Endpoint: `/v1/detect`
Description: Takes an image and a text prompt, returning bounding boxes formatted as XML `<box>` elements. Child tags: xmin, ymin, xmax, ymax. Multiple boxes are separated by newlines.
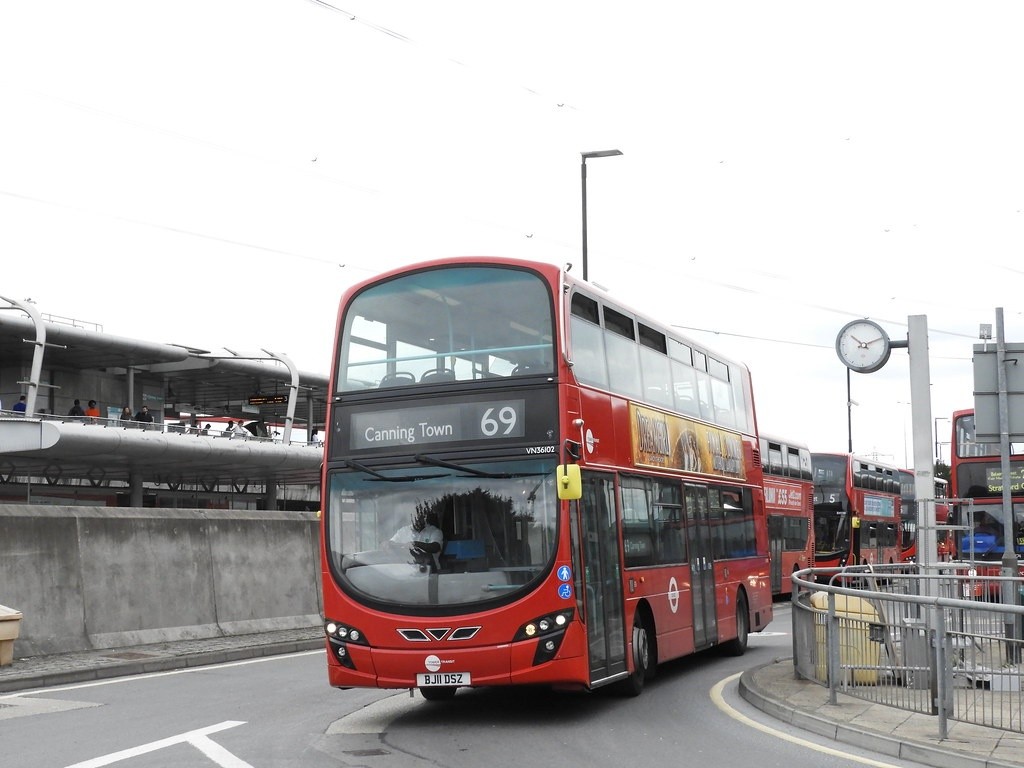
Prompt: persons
<box><xmin>194</xmin><ymin>420</ymin><xmax>248</xmax><ymax>439</ymax></box>
<box><xmin>12</xmin><ymin>397</ymin><xmax>26</xmax><ymax>414</ymax></box>
<box><xmin>379</xmin><ymin>503</ymin><xmax>443</xmax><ymax>571</ymax></box>
<box><xmin>311</xmin><ymin>430</ymin><xmax>320</xmax><ymax>446</ymax></box>
<box><xmin>69</xmin><ymin>399</ymin><xmax>100</xmax><ymax>425</ymax></box>
<box><xmin>120</xmin><ymin>406</ymin><xmax>153</xmax><ymax>429</ymax></box>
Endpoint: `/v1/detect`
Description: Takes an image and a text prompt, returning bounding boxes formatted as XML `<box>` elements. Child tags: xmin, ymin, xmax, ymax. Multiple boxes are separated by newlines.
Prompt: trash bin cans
<box><xmin>811</xmin><ymin>591</ymin><xmax>882</xmax><ymax>686</ymax></box>
<box><xmin>0</xmin><ymin>605</ymin><xmax>23</xmax><ymax>667</ymax></box>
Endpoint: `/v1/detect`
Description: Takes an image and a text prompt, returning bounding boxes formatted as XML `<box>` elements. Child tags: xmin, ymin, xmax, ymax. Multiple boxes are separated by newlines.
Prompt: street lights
<box><xmin>580</xmin><ymin>147</ymin><xmax>623</xmax><ymax>281</ymax></box>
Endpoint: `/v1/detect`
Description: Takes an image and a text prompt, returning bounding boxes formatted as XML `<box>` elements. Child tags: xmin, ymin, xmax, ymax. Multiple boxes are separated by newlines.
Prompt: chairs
<box><xmin>443</xmin><ymin>540</ymin><xmax>486</xmax><ymax>560</ymax></box>
<box><xmin>762</xmin><ymin>463</ymin><xmax>813</xmax><ymax>481</ymax></box>
<box><xmin>377</xmin><ymin>360</ymin><xmax>737</xmax><ymax>432</ymax></box>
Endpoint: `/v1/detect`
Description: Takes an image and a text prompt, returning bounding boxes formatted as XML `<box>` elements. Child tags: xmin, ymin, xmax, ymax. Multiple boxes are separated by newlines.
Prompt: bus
<box><xmin>948</xmin><ymin>409</ymin><xmax>1024</xmax><ymax>596</ymax></box>
<box><xmin>757</xmin><ymin>435</ymin><xmax>817</xmax><ymax>600</ymax></box>
<box><xmin>810</xmin><ymin>451</ymin><xmax>902</xmax><ymax>592</ymax></box>
<box><xmin>318</xmin><ymin>256</ymin><xmax>776</xmax><ymax>711</ymax></box>
<box><xmin>896</xmin><ymin>469</ymin><xmax>957</xmax><ymax>576</ymax></box>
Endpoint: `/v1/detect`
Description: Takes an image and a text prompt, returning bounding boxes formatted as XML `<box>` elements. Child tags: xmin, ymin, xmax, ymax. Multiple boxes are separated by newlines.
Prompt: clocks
<box><xmin>835</xmin><ymin>318</ymin><xmax>891</xmax><ymax>375</ymax></box>
